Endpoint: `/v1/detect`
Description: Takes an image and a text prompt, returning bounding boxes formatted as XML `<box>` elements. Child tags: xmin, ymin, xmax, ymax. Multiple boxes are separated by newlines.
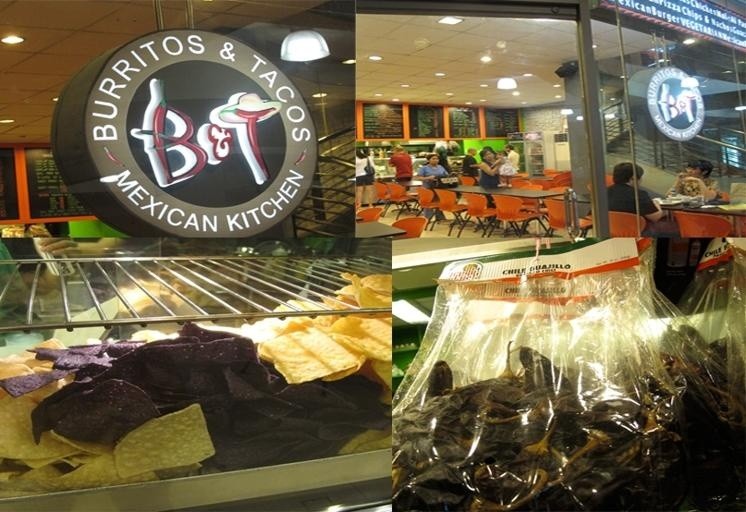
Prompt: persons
<box><xmin>588</xmin><ymin>161</ymin><xmax>674</xmax><ymax>288</ymax></box>
<box><xmin>387</xmin><ymin>144</ymin><xmax>414</xmax><ymax>192</ymax></box>
<box><xmin>461</xmin><ymin>148</ymin><xmax>481</xmax><ymax>177</ymax></box>
<box><xmin>496</xmin><ymin>150</ymin><xmax>517</xmax><ymax>188</ymax></box>
<box><xmin>480</xmin><ymin>147</ymin><xmax>506</xmax><ymax>192</ymax></box>
<box><xmin>414</xmin><ymin>153</ymin><xmax>450</xmax><ymax>226</ymax></box>
<box><xmin>504</xmin><ymin>143</ymin><xmax>520</xmax><ymax>171</ymax></box>
<box><xmin>433</xmin><ymin>137</ymin><xmax>460</xmax><ymax>174</ymax></box>
<box><xmin>665</xmin><ymin>153</ymin><xmax>724</xmax><ymax>207</ymax></box>
<box><xmin>355</xmin><ymin>143</ymin><xmax>376</xmax><ymax>215</ymax></box>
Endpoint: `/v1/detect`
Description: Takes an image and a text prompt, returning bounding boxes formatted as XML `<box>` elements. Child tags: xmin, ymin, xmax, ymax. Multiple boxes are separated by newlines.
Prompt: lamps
<box><xmin>496</xmin><ymin>77</ymin><xmax>518</xmax><ymax>89</ymax></box>
<box><xmin>280</xmin><ymin>30</ymin><xmax>331</xmax><ymax>62</ymax></box>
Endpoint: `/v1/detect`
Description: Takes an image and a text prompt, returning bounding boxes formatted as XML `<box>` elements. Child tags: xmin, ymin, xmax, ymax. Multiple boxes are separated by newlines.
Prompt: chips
<box><xmin>0</xmin><ymin>273</ymin><xmax>392</xmax><ymax>498</ymax></box>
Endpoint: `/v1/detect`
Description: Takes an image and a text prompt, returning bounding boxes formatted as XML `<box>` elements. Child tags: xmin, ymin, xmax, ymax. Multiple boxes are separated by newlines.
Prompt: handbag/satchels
<box><xmin>365</xmin><ymin>166</ymin><xmax>374</xmax><ymax>175</ymax></box>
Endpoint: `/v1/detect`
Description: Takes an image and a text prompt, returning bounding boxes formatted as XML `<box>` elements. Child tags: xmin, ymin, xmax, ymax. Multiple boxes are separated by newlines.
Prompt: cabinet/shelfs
<box><xmin>356</xmin><ymin>144</ymin><xmax>435</xmax><ymax>167</ymax></box>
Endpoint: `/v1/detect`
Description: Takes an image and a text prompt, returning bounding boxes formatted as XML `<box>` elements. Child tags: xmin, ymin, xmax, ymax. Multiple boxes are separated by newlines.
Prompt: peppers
<box><xmin>658</xmin><ymin>93</ymin><xmax>680</xmax><ymax>117</ymax></box>
<box><xmin>295</xmin><ymin>147</ymin><xmax>307</xmax><ymax>165</ymax></box>
<box><xmin>248</xmin><ymin>118</ymin><xmax>269</xmax><ymax>178</ymax></box>
<box><xmin>220</xmin><ymin>106</ymin><xmax>277</xmax><ymax>119</ymax></box>
<box><xmin>104</xmin><ymin>146</ymin><xmax>123</xmax><ymax>165</ymax></box>
<box><xmin>137</xmin><ymin>97</ymin><xmax>208</xmax><ymax>183</ymax></box>
<box><xmin>685</xmin><ymin>95</ymin><xmax>697</xmax><ymax>99</ymax></box>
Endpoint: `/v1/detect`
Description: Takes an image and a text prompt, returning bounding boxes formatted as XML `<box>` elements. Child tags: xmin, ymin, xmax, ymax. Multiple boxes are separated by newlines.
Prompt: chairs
<box><xmin>355</xmin><ymin>169</ymin><xmax>746</xmax><ymax>259</ymax></box>
